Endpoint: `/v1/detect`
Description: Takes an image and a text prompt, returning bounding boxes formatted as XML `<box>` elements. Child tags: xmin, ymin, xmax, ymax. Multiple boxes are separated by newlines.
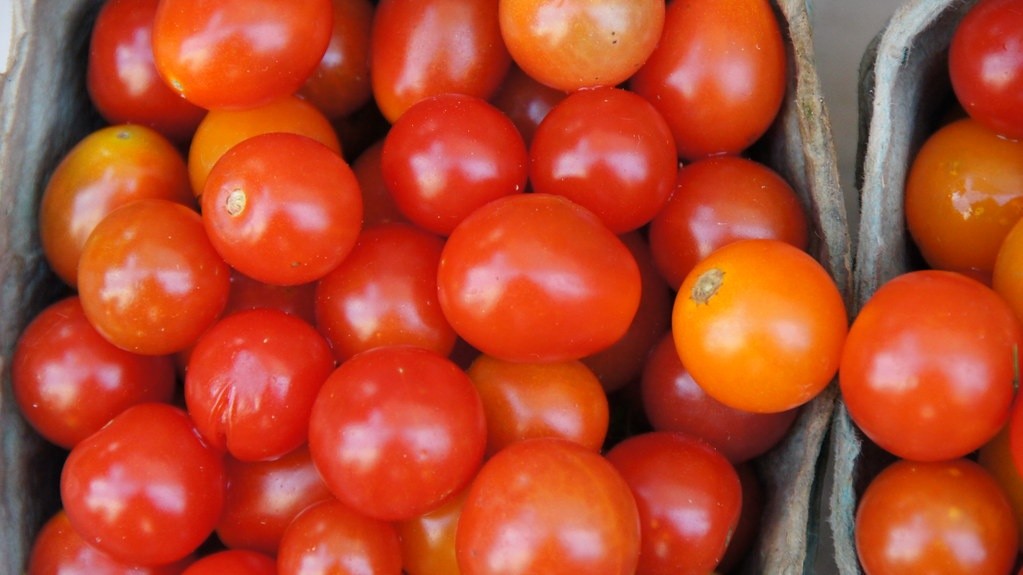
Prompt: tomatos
<box><xmin>0</xmin><ymin>0</ymin><xmax>1023</xmax><ymax>575</ymax></box>
